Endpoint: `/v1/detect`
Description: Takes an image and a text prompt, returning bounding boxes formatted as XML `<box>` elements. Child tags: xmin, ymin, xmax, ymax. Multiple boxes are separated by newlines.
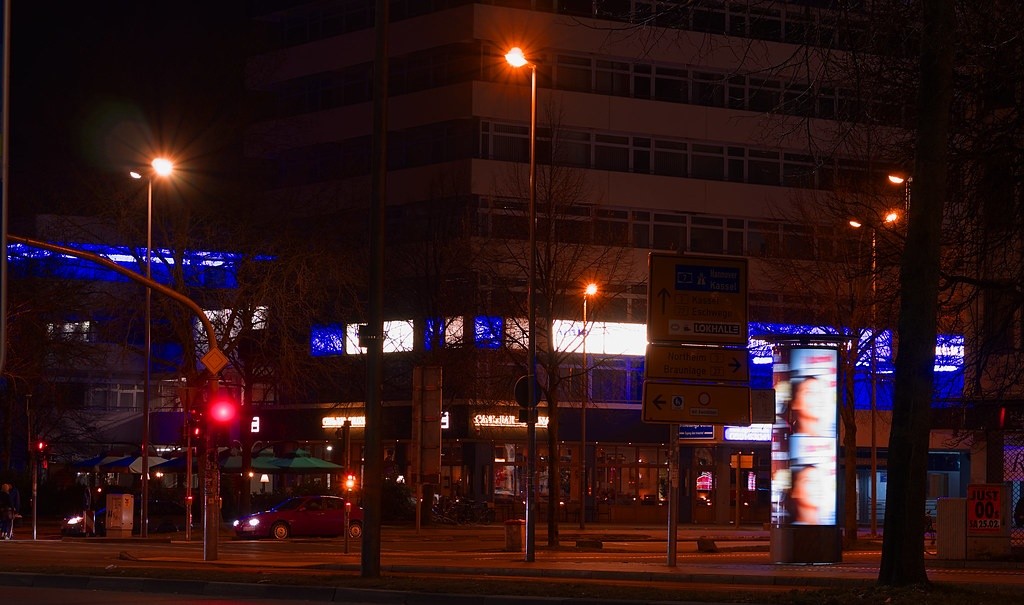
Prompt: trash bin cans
<box><xmin>503</xmin><ymin>519</ymin><xmax>526</xmax><ymax>552</ymax></box>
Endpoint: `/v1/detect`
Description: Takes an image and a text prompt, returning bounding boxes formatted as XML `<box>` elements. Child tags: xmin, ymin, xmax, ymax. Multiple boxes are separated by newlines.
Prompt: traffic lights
<box><xmin>345</xmin><ymin>474</ymin><xmax>356</xmax><ymax>491</ymax></box>
<box><xmin>179</xmin><ymin>386</ymin><xmax>204</xmax><ymax>443</ymax></box>
<box><xmin>204</xmin><ymin>392</ymin><xmax>239</xmax><ymax>454</ymax></box>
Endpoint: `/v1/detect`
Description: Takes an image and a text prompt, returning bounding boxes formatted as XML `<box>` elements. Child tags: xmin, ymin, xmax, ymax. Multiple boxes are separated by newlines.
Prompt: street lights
<box><xmin>139</xmin><ymin>152</ymin><xmax>173</xmax><ymax>537</ymax></box>
<box><xmin>504</xmin><ymin>46</ymin><xmax>538</xmax><ymax>561</ymax></box>
<box><xmin>848</xmin><ymin>219</ymin><xmax>878</xmax><ymax>537</ymax></box>
<box><xmin>31</xmin><ymin>439</ymin><xmax>47</xmax><ymax>539</ymax></box>
<box><xmin>579</xmin><ymin>282</ymin><xmax>597</xmax><ymax>529</ymax></box>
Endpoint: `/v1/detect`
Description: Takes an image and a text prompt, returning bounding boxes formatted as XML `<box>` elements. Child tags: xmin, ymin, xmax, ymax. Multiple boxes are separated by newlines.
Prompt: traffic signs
<box><xmin>642</xmin><ymin>252</ymin><xmax>751</xmax><ymax>425</ymax></box>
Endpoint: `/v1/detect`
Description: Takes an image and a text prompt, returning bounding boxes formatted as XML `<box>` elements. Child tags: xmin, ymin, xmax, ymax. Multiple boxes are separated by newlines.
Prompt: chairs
<box><xmin>487</xmin><ymin>503</ymin><xmax>548</xmax><ymax>523</ymax></box>
<box><xmin>645</xmin><ymin>495</ymin><xmax>656</xmax><ymax>504</ymax></box>
<box><xmin>597</xmin><ymin>503</ymin><xmax>611</xmax><ymax>522</ymax></box>
<box><xmin>565</xmin><ymin>503</ymin><xmax>577</xmax><ymax>522</ymax></box>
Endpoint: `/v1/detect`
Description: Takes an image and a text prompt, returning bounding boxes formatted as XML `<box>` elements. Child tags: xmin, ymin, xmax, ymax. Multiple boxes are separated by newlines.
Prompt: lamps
<box><xmin>260</xmin><ymin>474</ymin><xmax>269</xmax><ymax>492</ymax></box>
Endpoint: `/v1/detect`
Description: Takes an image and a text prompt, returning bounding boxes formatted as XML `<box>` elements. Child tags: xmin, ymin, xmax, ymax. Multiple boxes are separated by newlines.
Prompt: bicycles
<box><xmin>924</xmin><ymin>509</ymin><xmax>937</xmax><ymax>555</ymax></box>
<box><xmin>431</xmin><ymin>496</ymin><xmax>496</xmax><ymax>526</ymax></box>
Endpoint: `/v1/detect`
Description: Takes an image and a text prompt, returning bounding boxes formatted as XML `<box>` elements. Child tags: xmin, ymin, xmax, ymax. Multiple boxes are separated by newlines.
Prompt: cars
<box><xmin>232</xmin><ymin>495</ymin><xmax>363</xmax><ymax>540</ymax></box>
<box><xmin>61</xmin><ymin>498</ymin><xmax>193</xmax><ymax>536</ymax></box>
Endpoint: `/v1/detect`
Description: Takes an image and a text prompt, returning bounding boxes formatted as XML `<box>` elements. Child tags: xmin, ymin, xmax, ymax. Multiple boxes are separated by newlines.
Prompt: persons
<box><xmin>0</xmin><ymin>483</ymin><xmax>20</xmax><ymax>539</ymax></box>
